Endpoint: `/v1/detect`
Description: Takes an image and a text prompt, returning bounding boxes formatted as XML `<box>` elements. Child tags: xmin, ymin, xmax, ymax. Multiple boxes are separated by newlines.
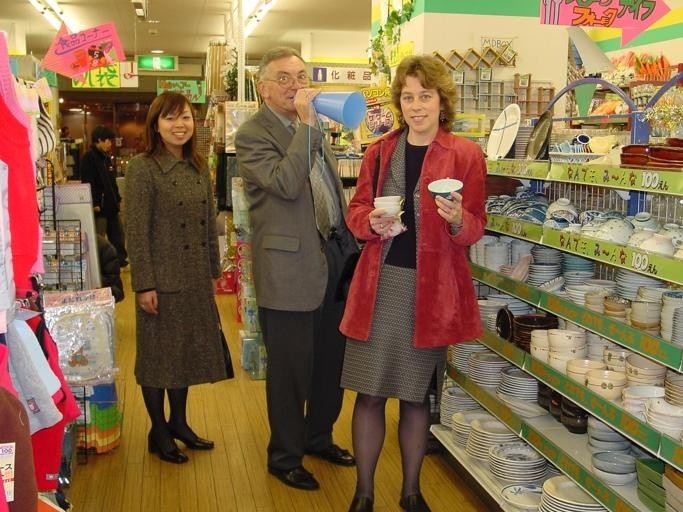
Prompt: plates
<box><xmin>515</xmin><ymin>126</ymin><xmax>534</xmax><ymax>159</ymax></box>
<box><xmin>439</xmin><ymin>339</ymin><xmax>604</xmax><ymax>512</ymax></box>
<box><xmin>553</xmin><ymin>140</ymin><xmax>592</xmax><ymax>152</ymax></box>
<box><xmin>478</xmin><ymin>238</ymin><xmax>682</xmax><ymax>346</ymax></box>
<box><xmin>486</xmin><ymin>104</ymin><xmax>521</xmax><ymax>160</ymax></box>
<box><xmin>526</xmin><ymin>111</ymin><xmax>552</xmax><ymax>161</ymax></box>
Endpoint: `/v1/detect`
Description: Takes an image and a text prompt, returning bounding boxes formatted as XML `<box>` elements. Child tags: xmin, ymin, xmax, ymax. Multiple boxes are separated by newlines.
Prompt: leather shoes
<box><xmin>348</xmin><ymin>496</ymin><xmax>374</xmax><ymax>512</ymax></box>
<box><xmin>307</xmin><ymin>442</ymin><xmax>355</xmax><ymax>466</ymax></box>
<box><xmin>269</xmin><ymin>464</ymin><xmax>319</xmax><ymax>490</ymax></box>
<box><xmin>399</xmin><ymin>493</ymin><xmax>430</xmax><ymax>511</ymax></box>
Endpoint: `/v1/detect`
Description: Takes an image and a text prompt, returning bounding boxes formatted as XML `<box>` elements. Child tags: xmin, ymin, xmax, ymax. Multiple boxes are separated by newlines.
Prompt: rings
<box><xmin>141</xmin><ymin>305</ymin><xmax>144</xmax><ymax>308</ymax></box>
<box><xmin>381</xmin><ymin>224</ymin><xmax>383</xmax><ymax>229</ymax></box>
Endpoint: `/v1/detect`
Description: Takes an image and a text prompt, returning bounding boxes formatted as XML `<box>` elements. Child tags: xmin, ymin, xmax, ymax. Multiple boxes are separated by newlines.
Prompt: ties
<box><xmin>291</xmin><ymin>120</ymin><xmax>331</xmax><ymax>241</ymax></box>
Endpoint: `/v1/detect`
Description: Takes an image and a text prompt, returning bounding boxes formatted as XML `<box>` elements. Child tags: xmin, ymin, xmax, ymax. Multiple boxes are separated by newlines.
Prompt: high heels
<box><xmin>173</xmin><ymin>425</ymin><xmax>214</xmax><ymax>450</ymax></box>
<box><xmin>148</xmin><ymin>435</ymin><xmax>189</xmax><ymax>464</ymax></box>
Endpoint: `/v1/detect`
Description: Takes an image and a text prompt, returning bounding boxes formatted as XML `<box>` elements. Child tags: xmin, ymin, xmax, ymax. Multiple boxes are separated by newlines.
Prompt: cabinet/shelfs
<box><xmin>429</xmin><ymin>108</ymin><xmax>683</xmax><ymax>511</ymax></box>
<box><xmin>441</xmin><ymin>68</ymin><xmax>515</xmax><ymax>113</ymax></box>
<box><xmin>515</xmin><ymin>74</ymin><xmax>555</xmax><ymax>118</ymax></box>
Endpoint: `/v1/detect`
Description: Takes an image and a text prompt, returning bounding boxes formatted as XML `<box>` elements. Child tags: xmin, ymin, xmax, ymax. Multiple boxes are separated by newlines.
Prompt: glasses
<box><xmin>265</xmin><ymin>77</ymin><xmax>312</xmax><ymax>90</ymax></box>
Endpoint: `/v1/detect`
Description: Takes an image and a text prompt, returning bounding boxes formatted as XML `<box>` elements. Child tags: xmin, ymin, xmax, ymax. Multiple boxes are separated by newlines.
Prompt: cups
<box><xmin>571</xmin><ymin>134</ymin><xmax>591</xmax><ymax>146</ymax></box>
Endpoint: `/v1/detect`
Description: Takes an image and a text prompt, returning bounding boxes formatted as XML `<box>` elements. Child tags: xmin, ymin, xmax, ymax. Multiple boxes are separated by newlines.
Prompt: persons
<box><xmin>80</xmin><ymin>128</ymin><xmax>128</xmax><ymax>268</ymax></box>
<box><xmin>340</xmin><ymin>55</ymin><xmax>487</xmax><ymax>512</ymax></box>
<box><xmin>98</xmin><ymin>236</ymin><xmax>124</xmax><ymax>303</ymax></box>
<box><xmin>235</xmin><ymin>47</ymin><xmax>355</xmax><ymax>489</ymax></box>
<box><xmin>124</xmin><ymin>92</ymin><xmax>233</xmax><ymax>464</ymax></box>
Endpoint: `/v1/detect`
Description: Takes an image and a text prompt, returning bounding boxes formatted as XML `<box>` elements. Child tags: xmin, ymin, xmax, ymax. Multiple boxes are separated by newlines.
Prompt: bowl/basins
<box><xmin>483</xmin><ymin>185</ymin><xmax>682</xmax><ymax>261</ymax></box>
<box><xmin>620</xmin><ymin>138</ymin><xmax>683</xmax><ymax>167</ymax></box>
<box><xmin>428</xmin><ymin>178</ymin><xmax>464</xmax><ymax>201</ymax></box>
<box><xmin>478</xmin><ymin>226</ymin><xmax>683</xmax><ymax>348</ymax></box>
<box><xmin>373</xmin><ymin>196</ymin><xmax>401</xmax><ymax>218</ymax></box>
<box><xmin>529</xmin><ymin>305</ymin><xmax>683</xmax><ymax>511</ymax></box>
